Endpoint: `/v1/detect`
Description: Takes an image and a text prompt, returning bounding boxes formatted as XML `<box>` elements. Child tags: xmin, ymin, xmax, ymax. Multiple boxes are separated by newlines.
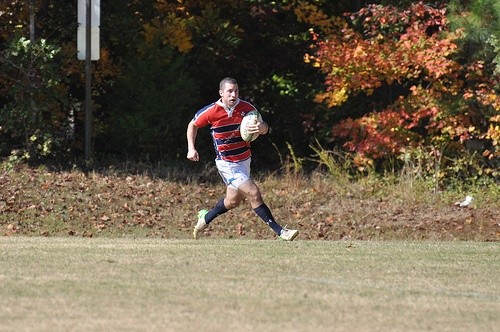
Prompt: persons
<box><xmin>185</xmin><ymin>77</ymin><xmax>299</xmax><ymax>242</ymax></box>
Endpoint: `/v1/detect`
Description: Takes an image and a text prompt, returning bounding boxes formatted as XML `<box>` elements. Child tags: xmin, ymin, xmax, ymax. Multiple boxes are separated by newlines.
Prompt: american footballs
<box><xmin>239</xmin><ymin>110</ymin><xmax>263</xmax><ymax>142</ymax></box>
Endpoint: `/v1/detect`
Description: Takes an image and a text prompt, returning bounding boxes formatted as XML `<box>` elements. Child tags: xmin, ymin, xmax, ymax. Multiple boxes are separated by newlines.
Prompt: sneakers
<box><xmin>277</xmin><ymin>226</ymin><xmax>299</xmax><ymax>241</ymax></box>
<box><xmin>193</xmin><ymin>209</ymin><xmax>209</xmax><ymax>239</ymax></box>
